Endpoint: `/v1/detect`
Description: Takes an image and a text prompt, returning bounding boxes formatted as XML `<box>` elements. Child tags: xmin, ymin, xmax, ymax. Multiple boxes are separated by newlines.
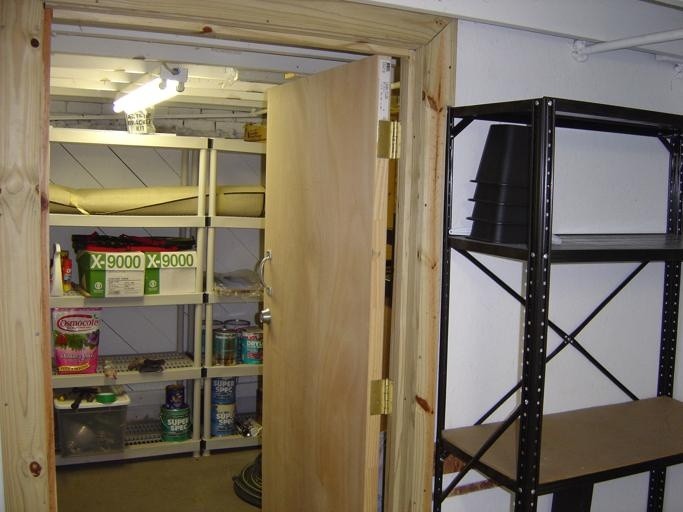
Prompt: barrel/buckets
<box><xmin>213</xmin><ymin>329</ymin><xmax>240</xmax><ymax>366</ymax></box>
<box><xmin>201</xmin><ymin>319</ymin><xmax>224</xmax><ymax>359</ymax></box>
<box><xmin>211</xmin><ymin>404</ymin><xmax>237</xmax><ymax>436</ymax></box>
<box><xmin>240</xmin><ymin>326</ymin><xmax>263</xmax><ymax>364</ymax></box>
<box><xmin>212</xmin><ymin>377</ymin><xmax>238</xmax><ymax>405</ymax></box>
<box><xmin>224</xmin><ymin>319</ymin><xmax>249</xmax><ymax>360</ymax></box>
<box><xmin>160</xmin><ymin>404</ymin><xmax>193</xmax><ymax>443</ymax></box>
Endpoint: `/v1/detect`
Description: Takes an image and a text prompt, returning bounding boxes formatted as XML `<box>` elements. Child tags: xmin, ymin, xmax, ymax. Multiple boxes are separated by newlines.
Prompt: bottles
<box><xmin>62</xmin><ymin>259</ymin><xmax>72</xmax><ymax>293</ymax></box>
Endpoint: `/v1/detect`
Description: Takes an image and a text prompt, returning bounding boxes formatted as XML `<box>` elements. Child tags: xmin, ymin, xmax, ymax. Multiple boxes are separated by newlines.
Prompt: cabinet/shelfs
<box><xmin>435</xmin><ymin>95</ymin><xmax>682</xmax><ymax>512</ymax></box>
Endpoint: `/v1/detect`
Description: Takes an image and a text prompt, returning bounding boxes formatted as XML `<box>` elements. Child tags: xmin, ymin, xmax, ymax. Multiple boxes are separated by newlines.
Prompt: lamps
<box><xmin>114</xmin><ymin>65</ymin><xmax>188</xmax><ymax>114</ymax></box>
